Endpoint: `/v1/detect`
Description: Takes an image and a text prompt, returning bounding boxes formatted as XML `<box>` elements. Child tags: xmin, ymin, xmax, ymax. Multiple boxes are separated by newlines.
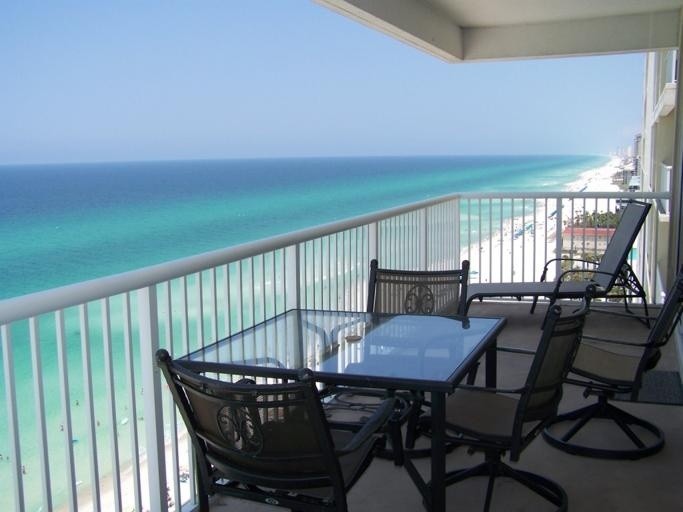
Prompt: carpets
<box><xmin>612</xmin><ymin>371</ymin><xmax>683</xmax><ymax>405</ymax></box>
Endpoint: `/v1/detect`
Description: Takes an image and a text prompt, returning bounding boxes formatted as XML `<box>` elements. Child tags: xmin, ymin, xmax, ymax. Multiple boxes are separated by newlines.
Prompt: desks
<box><xmin>174</xmin><ymin>308</ymin><xmax>507</xmax><ymax>512</ymax></box>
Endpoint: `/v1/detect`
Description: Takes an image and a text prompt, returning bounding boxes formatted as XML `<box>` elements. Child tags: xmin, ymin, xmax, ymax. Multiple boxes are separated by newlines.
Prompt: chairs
<box><xmin>540</xmin><ymin>264</ymin><xmax>683</xmax><ymax>457</ymax></box>
<box><xmin>462</xmin><ymin>198</ymin><xmax>654</xmax><ymax>329</ymax></box>
<box><xmin>365</xmin><ymin>258</ymin><xmax>482</xmax><ymax>452</ymax></box>
<box><xmin>156</xmin><ymin>350</ymin><xmax>401</xmax><ymax>512</ymax></box>
<box><xmin>418</xmin><ymin>283</ymin><xmax>596</xmax><ymax>512</ymax></box>
